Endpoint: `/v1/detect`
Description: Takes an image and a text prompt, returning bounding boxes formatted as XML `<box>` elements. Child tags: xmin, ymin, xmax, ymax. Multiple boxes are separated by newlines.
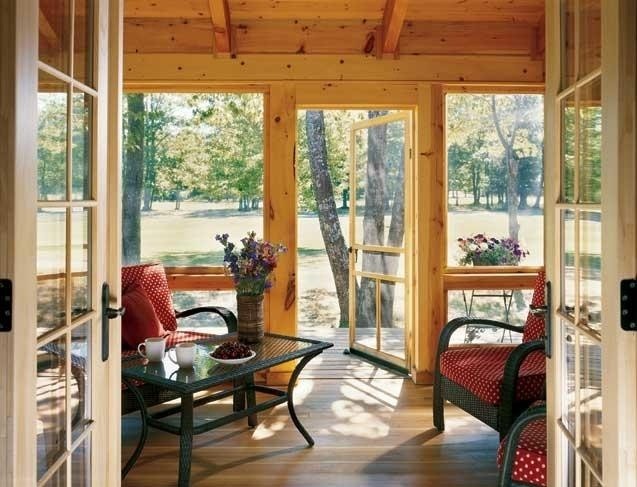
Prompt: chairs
<box><xmin>122</xmin><ymin>263</ymin><xmax>236</xmax><ymax>416</ymax></box>
<box><xmin>433</xmin><ymin>268</ymin><xmax>547</xmax><ymax>439</ymax></box>
<box><xmin>496</xmin><ymin>399</ymin><xmax>548</xmax><ymax>487</ymax></box>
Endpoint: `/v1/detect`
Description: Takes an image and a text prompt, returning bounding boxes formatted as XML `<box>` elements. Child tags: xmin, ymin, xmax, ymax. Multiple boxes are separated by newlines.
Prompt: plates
<box><xmin>207</xmin><ymin>350</ymin><xmax>256</xmax><ymax>365</ymax></box>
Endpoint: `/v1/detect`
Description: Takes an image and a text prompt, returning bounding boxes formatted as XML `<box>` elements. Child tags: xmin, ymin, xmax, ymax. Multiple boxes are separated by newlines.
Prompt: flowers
<box><xmin>215</xmin><ymin>232</ymin><xmax>288</xmax><ymax>296</ymax></box>
<box><xmin>457</xmin><ymin>232</ymin><xmax>531</xmax><ymax>266</ymax></box>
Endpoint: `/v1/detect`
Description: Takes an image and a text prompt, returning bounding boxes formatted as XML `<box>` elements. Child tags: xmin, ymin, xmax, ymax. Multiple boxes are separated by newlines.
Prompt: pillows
<box><xmin>124</xmin><ymin>283</ymin><xmax>163</xmax><ymax>346</ymax></box>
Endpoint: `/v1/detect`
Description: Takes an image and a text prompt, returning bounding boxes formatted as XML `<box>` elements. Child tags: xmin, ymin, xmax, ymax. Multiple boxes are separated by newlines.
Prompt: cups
<box><xmin>137</xmin><ymin>337</ymin><xmax>166</xmax><ymax>362</ymax></box>
<box><xmin>168</xmin><ymin>342</ymin><xmax>197</xmax><ymax>369</ymax></box>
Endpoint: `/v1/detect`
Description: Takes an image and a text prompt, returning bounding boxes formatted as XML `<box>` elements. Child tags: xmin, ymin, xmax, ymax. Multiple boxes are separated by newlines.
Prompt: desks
<box><xmin>121</xmin><ymin>333</ymin><xmax>334</xmax><ymax>487</ymax></box>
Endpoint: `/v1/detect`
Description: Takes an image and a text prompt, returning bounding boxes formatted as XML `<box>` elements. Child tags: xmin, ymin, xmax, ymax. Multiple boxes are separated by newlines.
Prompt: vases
<box><xmin>472</xmin><ymin>258</ymin><xmax>506</xmax><ymax>266</ymax></box>
<box><xmin>235</xmin><ymin>295</ymin><xmax>265</xmax><ymax>344</ymax></box>
